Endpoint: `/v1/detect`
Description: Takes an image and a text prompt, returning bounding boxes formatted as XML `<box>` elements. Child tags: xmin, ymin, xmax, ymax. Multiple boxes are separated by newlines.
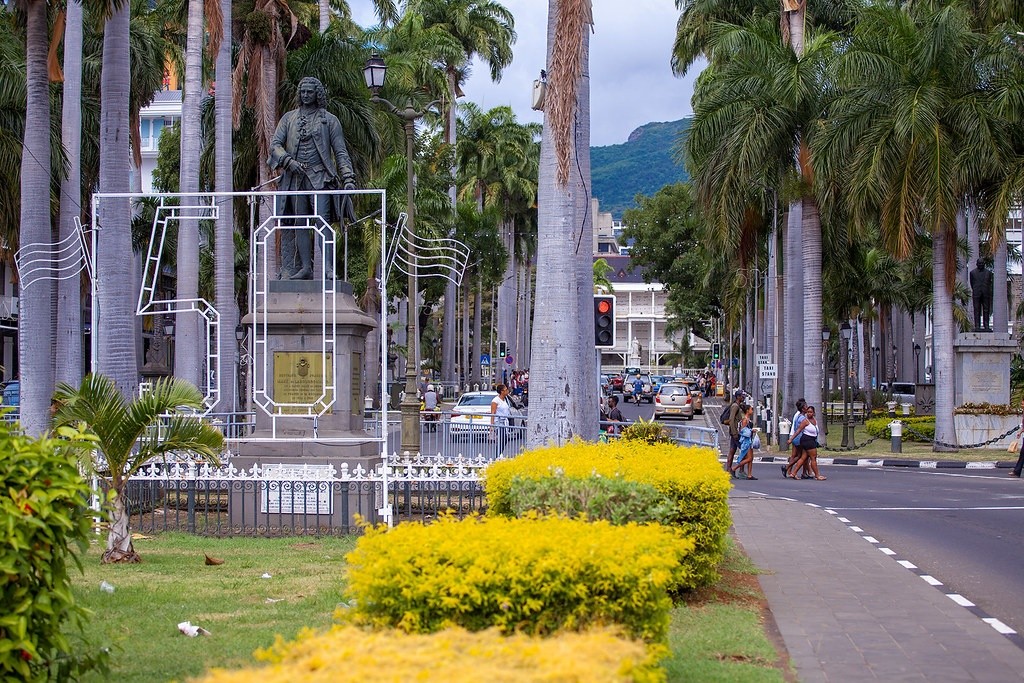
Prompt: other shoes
<box><xmin>634</xmin><ymin>400</ymin><xmax>636</xmax><ymax>404</ymax></box>
<box><xmin>802</xmin><ymin>474</ymin><xmax>813</xmax><ymax>479</ymax></box>
<box><xmin>781</xmin><ymin>465</ymin><xmax>787</xmax><ymax>478</ymax></box>
<box><xmin>748</xmin><ymin>476</ymin><xmax>758</xmax><ymax>480</ymax></box>
<box><xmin>736</xmin><ymin>471</ymin><xmax>747</xmax><ymax>479</ymax></box>
<box><xmin>1008</xmin><ymin>472</ymin><xmax>1020</xmax><ymax>477</ymax></box>
<box><xmin>729</xmin><ymin>468</ymin><xmax>737</xmax><ymax>479</ymax></box>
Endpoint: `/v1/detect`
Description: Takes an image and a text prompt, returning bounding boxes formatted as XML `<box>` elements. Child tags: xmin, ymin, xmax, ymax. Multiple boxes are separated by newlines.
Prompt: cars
<box><xmin>449</xmin><ymin>391</ymin><xmax>526</xmax><ymax>443</ymax></box>
<box><xmin>600</xmin><ymin>373</ymin><xmax>676</xmax><ymax>396</ymax></box>
<box><xmin>654</xmin><ymin>383</ymin><xmax>693</xmax><ymax>421</ymax></box>
<box><xmin>672</xmin><ymin>380</ymin><xmax>703</xmax><ymax>415</ymax></box>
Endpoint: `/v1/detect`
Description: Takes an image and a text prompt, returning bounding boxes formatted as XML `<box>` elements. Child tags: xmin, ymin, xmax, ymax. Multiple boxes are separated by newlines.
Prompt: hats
<box><xmin>735</xmin><ymin>390</ymin><xmax>747</xmax><ymax>396</ymax></box>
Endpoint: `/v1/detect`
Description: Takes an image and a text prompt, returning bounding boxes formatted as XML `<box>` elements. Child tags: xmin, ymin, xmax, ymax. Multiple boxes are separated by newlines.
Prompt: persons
<box><xmin>500</xmin><ymin>369</ymin><xmax>530</xmax><ymax>388</ymax></box>
<box><xmin>632</xmin><ymin>374</ymin><xmax>644</xmax><ymax>405</ymax></box>
<box><xmin>1008</xmin><ymin>410</ymin><xmax>1024</xmax><ymax>477</ymax></box>
<box><xmin>685</xmin><ymin>372</ymin><xmax>690</xmax><ymax>379</ymax></box>
<box><xmin>781</xmin><ymin>398</ymin><xmax>816</xmax><ymax>479</ymax></box>
<box><xmin>437</xmin><ymin>383</ymin><xmax>444</xmax><ymax>400</ymax></box>
<box><xmin>787</xmin><ymin>406</ymin><xmax>828</xmax><ymax>480</ymax></box>
<box><xmin>268</xmin><ymin>74</ymin><xmax>356</xmax><ymax>280</ymax></box>
<box><xmin>399</xmin><ymin>389</ymin><xmax>407</xmax><ymax>402</ymax></box>
<box><xmin>415</xmin><ymin>388</ymin><xmax>421</xmax><ymax>401</ymax></box>
<box><xmin>693</xmin><ymin>370</ymin><xmax>716</xmax><ymax>398</ymax></box>
<box><xmin>969</xmin><ymin>258</ymin><xmax>994</xmax><ymax>329</ymax></box>
<box><xmin>728</xmin><ymin>404</ymin><xmax>759</xmax><ymax>480</ymax></box>
<box><xmin>423</xmin><ymin>384</ymin><xmax>441</xmax><ymax>422</ymax></box>
<box><xmin>606</xmin><ymin>395</ymin><xmax>624</xmax><ymax>434</ymax></box>
<box><xmin>727</xmin><ymin>390</ymin><xmax>749</xmax><ymax>476</ymax></box>
<box><xmin>491</xmin><ymin>384</ymin><xmax>510</xmax><ymax>458</ymax></box>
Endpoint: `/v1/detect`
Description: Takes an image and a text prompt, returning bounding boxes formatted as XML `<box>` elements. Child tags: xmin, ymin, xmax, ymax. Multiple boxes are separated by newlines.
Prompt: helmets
<box><xmin>636</xmin><ymin>374</ymin><xmax>641</xmax><ymax>379</ymax></box>
<box><xmin>427</xmin><ymin>385</ymin><xmax>434</xmax><ymax>392</ymax></box>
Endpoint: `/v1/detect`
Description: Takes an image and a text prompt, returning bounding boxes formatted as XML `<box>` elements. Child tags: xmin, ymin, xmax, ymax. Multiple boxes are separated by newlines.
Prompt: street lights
<box><xmin>892</xmin><ymin>345</ymin><xmax>897</xmax><ymax>381</ymax></box>
<box><xmin>913</xmin><ymin>344</ymin><xmax>921</xmax><ymax>384</ymax></box>
<box><xmin>361</xmin><ymin>48</ymin><xmax>449</xmax><ymax>476</ymax></box>
<box><xmin>874</xmin><ymin>347</ymin><xmax>880</xmax><ymax>391</ymax></box>
<box><xmin>821</xmin><ymin>324</ymin><xmax>831</xmax><ymax>435</ymax></box>
<box><xmin>432</xmin><ymin>338</ymin><xmax>437</xmax><ymax>381</ymax></box>
<box><xmin>840</xmin><ymin>321</ymin><xmax>851</xmax><ymax>448</ymax></box>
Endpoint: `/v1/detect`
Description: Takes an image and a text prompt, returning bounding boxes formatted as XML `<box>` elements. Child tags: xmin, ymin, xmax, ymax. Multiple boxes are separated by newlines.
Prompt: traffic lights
<box><xmin>499</xmin><ymin>341</ymin><xmax>506</xmax><ymax>359</ymax></box>
<box><xmin>712</xmin><ymin>343</ymin><xmax>721</xmax><ymax>362</ymax></box>
<box><xmin>507</xmin><ymin>348</ymin><xmax>510</xmax><ymax>357</ymax></box>
<box><xmin>592</xmin><ymin>294</ymin><xmax>617</xmax><ymax>349</ymax></box>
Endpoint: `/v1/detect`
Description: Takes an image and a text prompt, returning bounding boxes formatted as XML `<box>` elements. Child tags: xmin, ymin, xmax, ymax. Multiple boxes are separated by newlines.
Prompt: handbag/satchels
<box><xmin>1007</xmin><ymin>438</ymin><xmax>1019</xmax><ymax>453</ymax></box>
<box><xmin>753</xmin><ymin>431</ymin><xmax>761</xmax><ymax>449</ymax></box>
<box><xmin>720</xmin><ymin>402</ymin><xmax>739</xmax><ymax>424</ymax></box>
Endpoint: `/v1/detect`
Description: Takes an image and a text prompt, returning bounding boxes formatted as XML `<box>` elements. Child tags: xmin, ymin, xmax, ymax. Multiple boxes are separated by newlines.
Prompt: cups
<box><xmin>100</xmin><ymin>580</ymin><xmax>114</xmax><ymax>593</ymax></box>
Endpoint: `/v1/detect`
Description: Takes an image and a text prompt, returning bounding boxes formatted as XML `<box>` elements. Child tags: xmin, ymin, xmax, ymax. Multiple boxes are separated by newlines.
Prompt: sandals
<box><xmin>815</xmin><ymin>476</ymin><xmax>827</xmax><ymax>480</ymax></box>
<box><xmin>790</xmin><ymin>473</ymin><xmax>801</xmax><ymax>480</ymax></box>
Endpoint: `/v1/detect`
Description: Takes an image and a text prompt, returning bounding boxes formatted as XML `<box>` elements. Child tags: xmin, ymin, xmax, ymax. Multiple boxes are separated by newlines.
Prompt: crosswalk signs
<box><xmin>480</xmin><ymin>354</ymin><xmax>489</xmax><ymax>365</ymax></box>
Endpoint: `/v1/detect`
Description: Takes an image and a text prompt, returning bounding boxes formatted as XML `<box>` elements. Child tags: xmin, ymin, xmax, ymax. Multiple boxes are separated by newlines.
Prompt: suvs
<box><xmin>423</xmin><ymin>402</ymin><xmax>443</xmax><ymax>433</ymax></box>
<box><xmin>622</xmin><ymin>374</ymin><xmax>656</xmax><ymax>404</ymax></box>
